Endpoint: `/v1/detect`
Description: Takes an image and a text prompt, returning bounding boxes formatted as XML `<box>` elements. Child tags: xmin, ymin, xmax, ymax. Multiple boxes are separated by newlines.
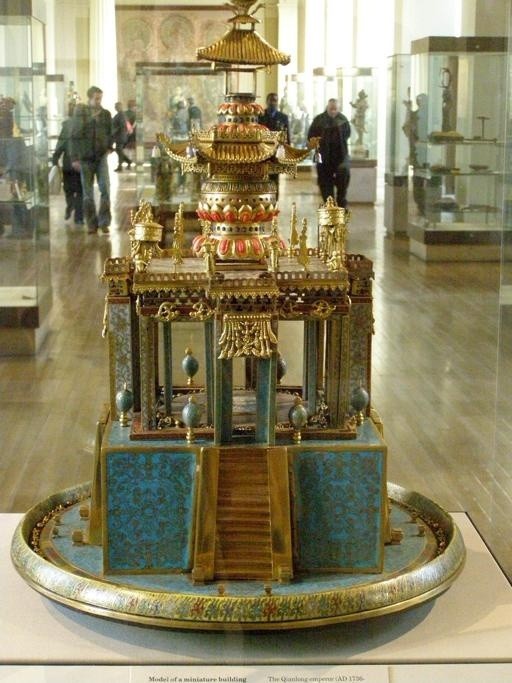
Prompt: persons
<box><xmin>66</xmin><ymin>84</ymin><xmax>116</xmax><ymax>236</ymax></box>
<box><xmin>185</xmin><ymin>96</ymin><xmax>202</xmax><ymax>133</ymax></box>
<box><xmin>400</xmin><ymin>92</ymin><xmax>434</xmax><ymax>214</ymax></box>
<box><xmin>35</xmin><ymin>107</ymin><xmax>49</xmax><ymax>154</ymax></box>
<box><xmin>307</xmin><ymin>97</ymin><xmax>352</xmax><ymax>211</ymax></box>
<box><xmin>172</xmin><ymin>100</ymin><xmax>190</xmax><ymax>136</ymax></box>
<box><xmin>257</xmin><ymin>91</ymin><xmax>290</xmax><ymax>151</ymax></box>
<box><xmin>123</xmin><ymin>99</ymin><xmax>138</xmax><ymax>147</ymax></box>
<box><xmin>0</xmin><ymin>96</ymin><xmax>17</xmax><ymax>138</ymax></box>
<box><xmin>348</xmin><ymin>89</ymin><xmax>369</xmax><ymax>145</ymax></box>
<box><xmin>51</xmin><ymin>101</ymin><xmax>86</xmax><ymax>227</ymax></box>
<box><xmin>107</xmin><ymin>101</ymin><xmax>133</xmax><ymax>172</ymax></box>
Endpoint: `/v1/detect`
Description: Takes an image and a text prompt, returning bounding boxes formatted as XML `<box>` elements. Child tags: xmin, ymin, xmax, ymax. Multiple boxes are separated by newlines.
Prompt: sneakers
<box><xmin>115</xmin><ymin>163</ymin><xmax>131</xmax><ymax>171</ymax></box>
<box><xmin>65</xmin><ymin>212</ymin><xmax>84</xmax><ymax>225</ymax></box>
<box><xmin>88</xmin><ymin>225</ymin><xmax>109</xmax><ymax>233</ymax></box>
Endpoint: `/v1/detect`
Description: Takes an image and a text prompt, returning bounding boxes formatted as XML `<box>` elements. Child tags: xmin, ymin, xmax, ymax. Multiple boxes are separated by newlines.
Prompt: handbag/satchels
<box><xmin>47</xmin><ymin>164</ymin><xmax>61</xmax><ymax>195</ymax></box>
